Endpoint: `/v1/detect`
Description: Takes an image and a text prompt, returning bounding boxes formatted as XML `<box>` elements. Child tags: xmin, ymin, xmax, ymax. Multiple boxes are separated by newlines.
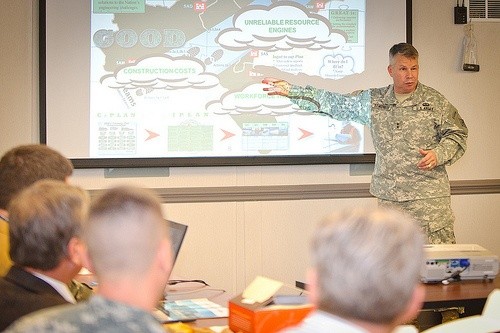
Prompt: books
<box><xmin>152</xmin><ymin>298</ymin><xmax>229</xmax><ymax>323</ymax></box>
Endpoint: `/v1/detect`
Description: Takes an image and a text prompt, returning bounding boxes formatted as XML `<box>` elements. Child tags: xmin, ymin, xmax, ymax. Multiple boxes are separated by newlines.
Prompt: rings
<box><xmin>429</xmin><ymin>166</ymin><xmax>433</xmax><ymax>170</ymax></box>
<box><xmin>428</xmin><ymin>163</ymin><xmax>431</xmax><ymax>167</ymax></box>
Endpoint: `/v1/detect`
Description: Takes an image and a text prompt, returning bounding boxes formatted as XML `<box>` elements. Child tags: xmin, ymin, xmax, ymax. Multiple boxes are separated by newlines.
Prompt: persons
<box><xmin>277</xmin><ymin>203</ymin><xmax>428</xmax><ymax>333</ymax></box>
<box><xmin>0</xmin><ymin>179</ymin><xmax>91</xmax><ymax>333</ymax></box>
<box><xmin>1</xmin><ymin>184</ymin><xmax>175</xmax><ymax>333</ymax></box>
<box><xmin>262</xmin><ymin>42</ymin><xmax>468</xmax><ymax>244</ymax></box>
<box><xmin>0</xmin><ymin>145</ymin><xmax>73</xmax><ymax>276</ymax></box>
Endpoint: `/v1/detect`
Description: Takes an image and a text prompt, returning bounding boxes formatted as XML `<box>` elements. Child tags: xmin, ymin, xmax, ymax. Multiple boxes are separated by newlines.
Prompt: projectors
<box><xmin>421</xmin><ymin>244</ymin><xmax>499</xmax><ymax>285</ymax></box>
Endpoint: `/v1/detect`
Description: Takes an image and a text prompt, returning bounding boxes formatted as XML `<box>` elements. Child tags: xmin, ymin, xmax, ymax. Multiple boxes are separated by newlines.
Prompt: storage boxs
<box><xmin>228</xmin><ymin>275</ymin><xmax>316</xmax><ymax>333</ymax></box>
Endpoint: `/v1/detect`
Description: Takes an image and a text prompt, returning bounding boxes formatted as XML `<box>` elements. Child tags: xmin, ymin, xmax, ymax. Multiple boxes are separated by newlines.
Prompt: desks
<box><xmin>73</xmin><ymin>272</ymin><xmax>232</xmax><ymax>327</ymax></box>
<box><xmin>296</xmin><ymin>279</ymin><xmax>500</xmax><ymax>323</ymax></box>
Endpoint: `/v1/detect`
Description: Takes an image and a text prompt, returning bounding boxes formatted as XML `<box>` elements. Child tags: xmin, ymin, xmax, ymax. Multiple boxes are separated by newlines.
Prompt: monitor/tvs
<box><xmin>164</xmin><ymin>218</ymin><xmax>188</xmax><ymax>264</ymax></box>
